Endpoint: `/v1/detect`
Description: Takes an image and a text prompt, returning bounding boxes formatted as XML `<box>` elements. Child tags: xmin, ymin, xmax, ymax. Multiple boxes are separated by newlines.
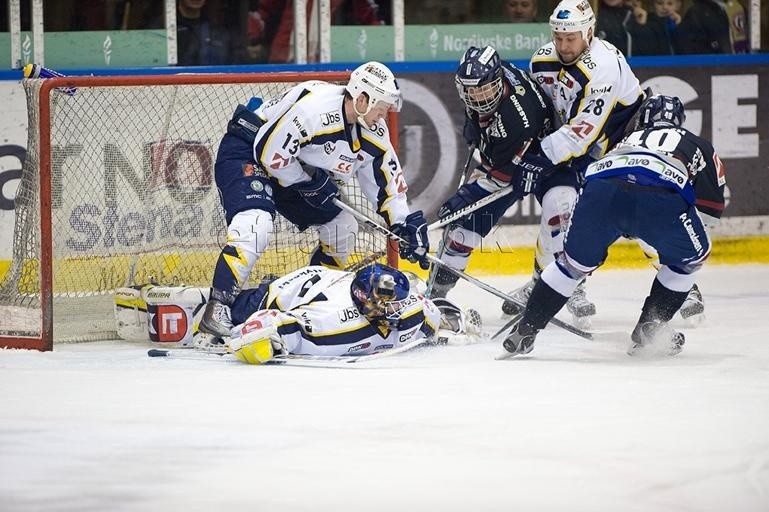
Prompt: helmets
<box><xmin>454</xmin><ymin>46</ymin><xmax>504</xmax><ymax>113</ymax></box>
<box><xmin>548</xmin><ymin>0</ymin><xmax>597</xmax><ymax>66</ymax></box>
<box><xmin>346</xmin><ymin>60</ymin><xmax>403</xmax><ymax>130</ymax></box>
<box><xmin>351</xmin><ymin>263</ymin><xmax>411</xmax><ymax>328</ymax></box>
<box><xmin>634</xmin><ymin>94</ymin><xmax>684</xmax><ymax>131</ymax></box>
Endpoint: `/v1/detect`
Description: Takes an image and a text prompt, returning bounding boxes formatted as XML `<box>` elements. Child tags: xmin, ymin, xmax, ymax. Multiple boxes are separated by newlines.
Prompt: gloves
<box><xmin>509</xmin><ymin>151</ymin><xmax>551</xmax><ymax>201</ymax></box>
<box><xmin>385</xmin><ymin>210</ymin><xmax>431</xmax><ymax>270</ymax></box>
<box><xmin>291</xmin><ymin>169</ymin><xmax>340</xmax><ymax>213</ymax></box>
<box><xmin>460</xmin><ymin>117</ymin><xmax>480</xmax><ymax>150</ymax></box>
<box><xmin>437</xmin><ymin>184</ymin><xmax>471</xmax><ymax>218</ymax></box>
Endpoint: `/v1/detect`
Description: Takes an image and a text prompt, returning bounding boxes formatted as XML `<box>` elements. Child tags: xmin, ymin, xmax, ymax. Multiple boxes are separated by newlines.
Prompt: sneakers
<box><xmin>565</xmin><ymin>278</ymin><xmax>598</xmax><ymax>317</ymax></box>
<box><xmin>630</xmin><ymin>306</ymin><xmax>685</xmax><ymax>348</ymax></box>
<box><xmin>199</xmin><ymin>297</ymin><xmax>237</xmax><ymax>340</ymax></box>
<box><xmin>502</xmin><ymin>281</ymin><xmax>537</xmax><ymax>357</ymax></box>
<box><xmin>679</xmin><ymin>284</ymin><xmax>705</xmax><ymax>318</ymax></box>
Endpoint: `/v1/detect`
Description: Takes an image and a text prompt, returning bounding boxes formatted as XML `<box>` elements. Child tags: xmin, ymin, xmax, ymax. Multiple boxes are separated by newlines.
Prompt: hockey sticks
<box><xmin>490</xmin><ymin>308</ymin><xmax>527</xmax><ymax>340</ymax></box>
<box><xmin>149</xmin><ymin>338</ymin><xmax>426</xmax><ymax>364</ymax></box>
<box><xmin>332</xmin><ymin>198</ymin><xmax>631</xmax><ymax>346</ymax></box>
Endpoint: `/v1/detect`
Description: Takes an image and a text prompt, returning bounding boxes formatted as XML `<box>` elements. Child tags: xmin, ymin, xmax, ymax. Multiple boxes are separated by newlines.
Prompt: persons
<box><xmin>0</xmin><ymin>0</ymin><xmax>748</xmax><ymax>65</ymax></box>
<box><xmin>503</xmin><ymin>94</ymin><xmax>731</xmax><ymax>358</ymax></box>
<box><xmin>503</xmin><ymin>0</ymin><xmax>703</xmax><ymax>318</ymax></box>
<box><xmin>199</xmin><ymin>61</ymin><xmax>429</xmax><ymax>339</ymax></box>
<box><xmin>426</xmin><ymin>47</ymin><xmax>596</xmax><ymax>317</ymax></box>
